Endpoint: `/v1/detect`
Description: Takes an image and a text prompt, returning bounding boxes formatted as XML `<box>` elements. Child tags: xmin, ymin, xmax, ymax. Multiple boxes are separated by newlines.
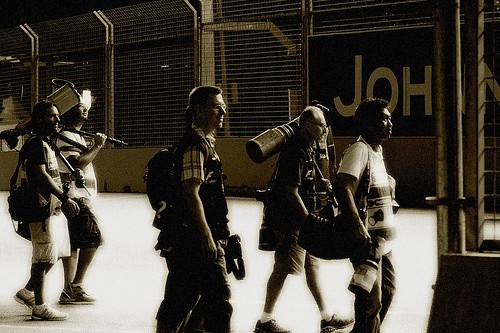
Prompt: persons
<box><xmin>254</xmin><ymin>106</ymin><xmax>354</xmax><ymax>333</ymax></box>
<box><xmin>156</xmin><ymin>85</ymin><xmax>234</xmax><ymax>333</ymax></box>
<box><xmin>56</xmin><ymin>100</ymin><xmax>107</xmax><ymax>303</ymax></box>
<box><xmin>333</xmin><ymin>100</ymin><xmax>397</xmax><ymax>333</ymax></box>
<box><xmin>15</xmin><ymin>106</ymin><xmax>80</xmax><ymax>321</ymax></box>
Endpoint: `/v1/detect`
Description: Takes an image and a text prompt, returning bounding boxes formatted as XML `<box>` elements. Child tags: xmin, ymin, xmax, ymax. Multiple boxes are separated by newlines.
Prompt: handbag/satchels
<box><xmin>15</xmin><ymin>222</ymin><xmax>33</xmax><ymax>241</ymax></box>
<box><xmin>224</xmin><ymin>234</ymin><xmax>242</xmax><ymax>259</ymax></box>
<box><xmin>260</xmin><ymin>229</ymin><xmax>279</xmax><ymax>250</ymax></box>
<box><xmin>175</xmin><ymin>182</ymin><xmax>228</xmax><ymax>254</ymax></box>
<box><xmin>297</xmin><ymin>207</ymin><xmax>365</xmax><ymax>260</ymax></box>
<box><xmin>6</xmin><ymin>181</ymin><xmax>54</xmax><ymax>223</ymax></box>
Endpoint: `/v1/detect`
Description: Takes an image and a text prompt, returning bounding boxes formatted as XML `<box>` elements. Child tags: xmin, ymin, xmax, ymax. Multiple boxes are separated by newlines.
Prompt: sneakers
<box><xmin>254</xmin><ymin>320</ymin><xmax>292</xmax><ymax>333</ymax></box>
<box><xmin>320</xmin><ymin>315</ymin><xmax>354</xmax><ymax>333</ymax></box>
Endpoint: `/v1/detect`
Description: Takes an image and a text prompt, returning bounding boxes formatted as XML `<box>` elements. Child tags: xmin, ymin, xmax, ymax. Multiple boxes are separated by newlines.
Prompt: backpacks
<box><xmin>146</xmin><ymin>146</ymin><xmax>183</xmax><ymax>213</ymax></box>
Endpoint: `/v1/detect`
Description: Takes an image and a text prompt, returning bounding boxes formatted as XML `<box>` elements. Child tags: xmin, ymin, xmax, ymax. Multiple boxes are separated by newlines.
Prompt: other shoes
<box><xmin>58</xmin><ymin>282</ymin><xmax>96</xmax><ymax>303</ymax></box>
<box><xmin>16</xmin><ymin>289</ymin><xmax>35</xmax><ymax>310</ymax></box>
<box><xmin>33</xmin><ymin>305</ymin><xmax>65</xmax><ymax>322</ymax></box>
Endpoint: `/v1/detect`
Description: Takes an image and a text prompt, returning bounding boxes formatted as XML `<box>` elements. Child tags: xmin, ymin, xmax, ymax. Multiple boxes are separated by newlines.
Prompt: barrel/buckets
<box><xmin>347</xmin><ymin>247</ymin><xmax>382</xmax><ymax>297</ymax></box>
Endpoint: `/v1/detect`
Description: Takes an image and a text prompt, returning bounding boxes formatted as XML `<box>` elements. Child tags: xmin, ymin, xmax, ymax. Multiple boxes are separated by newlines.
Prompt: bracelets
<box><xmin>58</xmin><ymin>193</ymin><xmax>69</xmax><ymax>203</ymax></box>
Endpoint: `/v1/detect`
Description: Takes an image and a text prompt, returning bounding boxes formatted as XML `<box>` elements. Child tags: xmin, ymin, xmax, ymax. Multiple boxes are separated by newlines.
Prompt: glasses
<box><xmin>194</xmin><ymin>103</ymin><xmax>227</xmax><ymax>111</ymax></box>
<box><xmin>311</xmin><ymin>122</ymin><xmax>329</xmax><ymax>128</ymax></box>
